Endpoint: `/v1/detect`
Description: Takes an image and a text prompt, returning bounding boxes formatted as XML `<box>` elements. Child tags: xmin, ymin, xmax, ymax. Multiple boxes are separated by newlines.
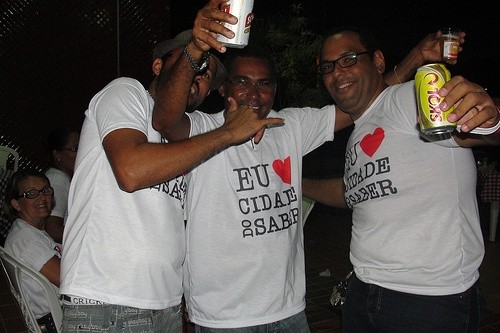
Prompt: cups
<box><xmin>439</xmin><ymin>25</ymin><xmax>461</xmax><ymax>65</ymax></box>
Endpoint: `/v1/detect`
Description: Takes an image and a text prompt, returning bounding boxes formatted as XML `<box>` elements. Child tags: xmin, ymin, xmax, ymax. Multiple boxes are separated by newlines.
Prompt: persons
<box><xmin>3</xmin><ymin>0</ymin><xmax>500</xmax><ymax>333</ymax></box>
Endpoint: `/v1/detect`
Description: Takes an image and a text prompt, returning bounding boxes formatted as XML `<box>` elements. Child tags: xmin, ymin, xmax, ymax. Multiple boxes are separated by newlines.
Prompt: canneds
<box><xmin>415</xmin><ymin>63</ymin><xmax>458</xmax><ymax>135</ymax></box>
<box><xmin>215</xmin><ymin>0</ymin><xmax>254</xmax><ymax>48</ymax></box>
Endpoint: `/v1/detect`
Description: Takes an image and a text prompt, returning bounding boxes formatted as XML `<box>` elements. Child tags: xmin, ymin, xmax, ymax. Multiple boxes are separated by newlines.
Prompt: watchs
<box><xmin>183</xmin><ymin>41</ymin><xmax>209</xmax><ymax>72</ymax></box>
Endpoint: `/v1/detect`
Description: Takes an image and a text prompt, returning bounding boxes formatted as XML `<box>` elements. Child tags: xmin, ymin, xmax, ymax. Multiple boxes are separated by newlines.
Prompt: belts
<box><xmin>61</xmin><ymin>294</ymin><xmax>108</xmax><ymax>305</ymax></box>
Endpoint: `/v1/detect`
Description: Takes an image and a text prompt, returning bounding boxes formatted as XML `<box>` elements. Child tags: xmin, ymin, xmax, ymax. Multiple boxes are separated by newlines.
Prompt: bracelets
<box><xmin>393</xmin><ymin>65</ymin><xmax>402</xmax><ymax>85</ymax></box>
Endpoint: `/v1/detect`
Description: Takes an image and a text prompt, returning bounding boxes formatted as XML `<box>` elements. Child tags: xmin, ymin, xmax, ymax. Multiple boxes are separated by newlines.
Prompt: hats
<box><xmin>152</xmin><ymin>29</ymin><xmax>227</xmax><ymax>91</ymax></box>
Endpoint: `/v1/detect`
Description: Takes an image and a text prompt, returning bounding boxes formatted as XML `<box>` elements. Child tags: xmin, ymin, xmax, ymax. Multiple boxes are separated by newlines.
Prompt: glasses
<box><xmin>226</xmin><ymin>77</ymin><xmax>272</xmax><ymax>90</ymax></box>
<box><xmin>315</xmin><ymin>52</ymin><xmax>369</xmax><ymax>76</ymax></box>
<box><xmin>16</xmin><ymin>187</ymin><xmax>53</xmax><ymax>199</ymax></box>
<box><xmin>53</xmin><ymin>147</ymin><xmax>78</xmax><ymax>152</ymax></box>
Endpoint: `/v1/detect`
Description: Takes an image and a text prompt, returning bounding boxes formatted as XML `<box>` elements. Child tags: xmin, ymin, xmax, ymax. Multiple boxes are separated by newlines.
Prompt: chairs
<box><xmin>0</xmin><ymin>246</ymin><xmax>63</xmax><ymax>333</ymax></box>
<box><xmin>0</xmin><ymin>145</ymin><xmax>20</xmax><ymax>172</ymax></box>
